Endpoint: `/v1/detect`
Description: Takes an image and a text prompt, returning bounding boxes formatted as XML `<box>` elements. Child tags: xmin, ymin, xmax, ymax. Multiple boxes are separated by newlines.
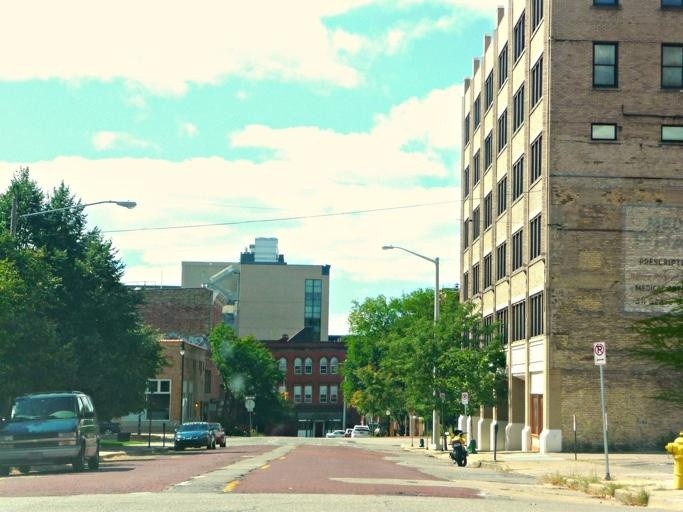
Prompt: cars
<box><xmin>174</xmin><ymin>422</ymin><xmax>226</xmax><ymax>450</ymax></box>
<box><xmin>326</xmin><ymin>422</ymin><xmax>387</xmax><ymax>438</ymax></box>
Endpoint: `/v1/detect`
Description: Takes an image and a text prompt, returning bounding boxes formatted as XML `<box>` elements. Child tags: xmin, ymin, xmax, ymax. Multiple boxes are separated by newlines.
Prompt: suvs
<box><xmin>0</xmin><ymin>390</ymin><xmax>101</xmax><ymax>478</ymax></box>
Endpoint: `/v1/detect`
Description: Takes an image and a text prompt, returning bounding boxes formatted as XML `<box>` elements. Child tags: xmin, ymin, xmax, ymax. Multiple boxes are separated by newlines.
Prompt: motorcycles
<box><xmin>444</xmin><ymin>430</ymin><xmax>469</xmax><ymax>466</ymax></box>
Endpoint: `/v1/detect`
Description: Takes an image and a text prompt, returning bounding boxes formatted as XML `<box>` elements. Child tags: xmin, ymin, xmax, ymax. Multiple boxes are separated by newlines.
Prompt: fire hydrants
<box><xmin>666</xmin><ymin>431</ymin><xmax>682</xmax><ymax>491</ymax></box>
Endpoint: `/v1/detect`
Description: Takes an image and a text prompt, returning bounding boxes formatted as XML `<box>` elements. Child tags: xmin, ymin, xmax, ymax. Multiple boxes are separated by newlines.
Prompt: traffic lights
<box><xmin>194</xmin><ymin>401</ymin><xmax>199</xmax><ymax>408</ymax></box>
<box><xmin>281</xmin><ymin>392</ymin><xmax>285</xmax><ymax>400</ymax></box>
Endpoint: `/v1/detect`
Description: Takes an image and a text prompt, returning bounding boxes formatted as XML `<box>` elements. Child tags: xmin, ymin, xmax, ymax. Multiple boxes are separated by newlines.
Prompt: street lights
<box><xmin>383</xmin><ymin>245</ymin><xmax>440</xmax><ymax>445</ymax></box>
<box><xmin>177</xmin><ymin>341</ymin><xmax>185</xmax><ymax>426</ymax></box>
<box><xmin>9</xmin><ymin>198</ymin><xmax>136</xmax><ymax>239</ymax></box>
